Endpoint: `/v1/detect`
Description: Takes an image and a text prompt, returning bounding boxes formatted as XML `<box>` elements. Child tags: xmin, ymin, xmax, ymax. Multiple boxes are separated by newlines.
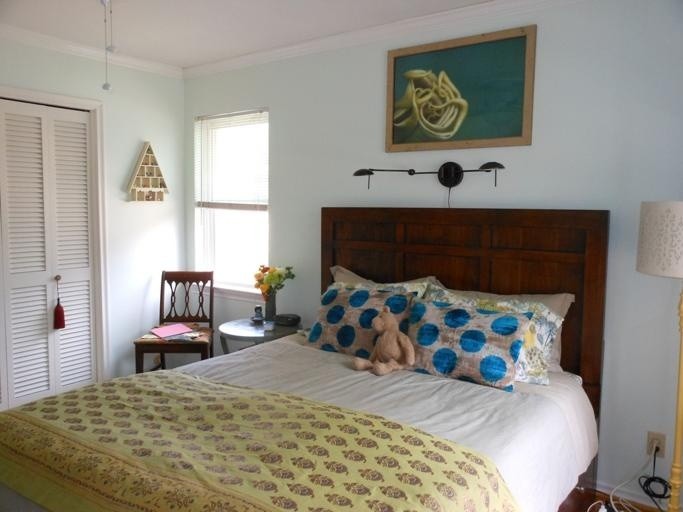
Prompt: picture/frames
<box><xmin>382</xmin><ymin>23</ymin><xmax>537</xmax><ymax>150</ymax></box>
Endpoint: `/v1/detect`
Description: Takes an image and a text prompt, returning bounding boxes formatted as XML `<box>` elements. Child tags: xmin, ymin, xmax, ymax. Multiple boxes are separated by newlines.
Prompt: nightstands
<box><xmin>218</xmin><ymin>317</ymin><xmax>301</xmax><ymax>354</ymax></box>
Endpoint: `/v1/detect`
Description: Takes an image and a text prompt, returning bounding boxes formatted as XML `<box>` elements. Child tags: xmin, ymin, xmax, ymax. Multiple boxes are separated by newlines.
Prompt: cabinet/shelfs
<box><xmin>126</xmin><ymin>142</ymin><xmax>170</xmax><ymax>202</ymax></box>
<box><xmin>0</xmin><ymin>86</ymin><xmax>105</xmax><ymax>407</ymax></box>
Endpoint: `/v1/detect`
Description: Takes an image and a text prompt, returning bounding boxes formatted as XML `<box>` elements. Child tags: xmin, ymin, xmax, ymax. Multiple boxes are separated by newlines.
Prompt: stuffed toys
<box><xmin>349</xmin><ymin>305</ymin><xmax>414</xmax><ymax>377</ymax></box>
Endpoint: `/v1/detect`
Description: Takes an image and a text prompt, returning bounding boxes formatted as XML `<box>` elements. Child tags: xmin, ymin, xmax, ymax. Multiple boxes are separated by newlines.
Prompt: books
<box><xmin>150</xmin><ymin>322</ymin><xmax>191</xmax><ymax>338</ymax></box>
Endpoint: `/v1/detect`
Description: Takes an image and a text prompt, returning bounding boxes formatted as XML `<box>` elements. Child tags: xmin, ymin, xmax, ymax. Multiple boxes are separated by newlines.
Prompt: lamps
<box><xmin>349</xmin><ymin>161</ymin><xmax>503</xmax><ymax>192</ymax></box>
<box><xmin>634</xmin><ymin>193</ymin><xmax>683</xmax><ymax>511</ymax></box>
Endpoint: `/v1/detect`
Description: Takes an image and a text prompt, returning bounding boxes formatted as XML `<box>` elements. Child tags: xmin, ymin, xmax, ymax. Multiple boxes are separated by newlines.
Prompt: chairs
<box><xmin>133</xmin><ymin>267</ymin><xmax>214</xmax><ymax>370</ymax></box>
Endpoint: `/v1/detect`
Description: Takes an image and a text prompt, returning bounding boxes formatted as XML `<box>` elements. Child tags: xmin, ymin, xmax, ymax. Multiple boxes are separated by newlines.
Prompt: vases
<box><xmin>263</xmin><ymin>298</ymin><xmax>276</xmax><ymax>320</ymax></box>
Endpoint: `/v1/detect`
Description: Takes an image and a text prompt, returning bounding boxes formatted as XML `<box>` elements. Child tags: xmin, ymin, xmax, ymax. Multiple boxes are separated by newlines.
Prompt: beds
<box><xmin>1</xmin><ymin>207</ymin><xmax>608</xmax><ymax>510</ymax></box>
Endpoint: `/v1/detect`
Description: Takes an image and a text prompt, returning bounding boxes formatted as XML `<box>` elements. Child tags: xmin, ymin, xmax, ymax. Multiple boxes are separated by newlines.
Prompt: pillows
<box><xmin>304</xmin><ymin>264</ymin><xmax>574</xmax><ymax>392</ymax></box>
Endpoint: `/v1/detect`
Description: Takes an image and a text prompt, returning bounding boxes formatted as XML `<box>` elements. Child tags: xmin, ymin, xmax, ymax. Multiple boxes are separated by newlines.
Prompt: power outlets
<box><xmin>644</xmin><ymin>428</ymin><xmax>668</xmax><ymax>459</ymax></box>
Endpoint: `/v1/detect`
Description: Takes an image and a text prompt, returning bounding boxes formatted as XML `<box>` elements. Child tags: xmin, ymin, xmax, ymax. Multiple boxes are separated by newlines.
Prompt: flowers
<box><xmin>253</xmin><ymin>260</ymin><xmax>295</xmax><ymax>301</ymax></box>
